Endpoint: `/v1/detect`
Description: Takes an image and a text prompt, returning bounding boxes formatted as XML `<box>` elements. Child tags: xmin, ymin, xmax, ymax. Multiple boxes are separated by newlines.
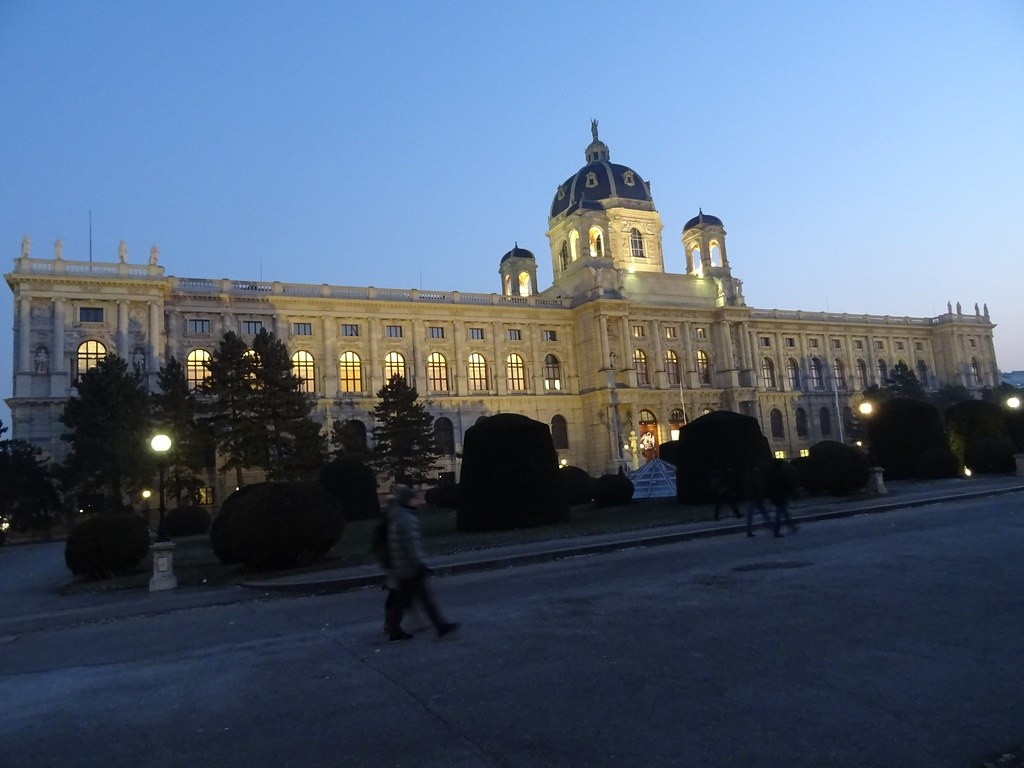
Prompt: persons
<box><xmin>373</xmin><ymin>483</ymin><xmax>462</xmax><ymax>644</ymax></box>
<box><xmin>747</xmin><ymin>455</ymin><xmax>800</xmax><ymax>538</ymax></box>
<box><xmin>706</xmin><ymin>456</ymin><xmax>742</xmax><ymax>521</ymax></box>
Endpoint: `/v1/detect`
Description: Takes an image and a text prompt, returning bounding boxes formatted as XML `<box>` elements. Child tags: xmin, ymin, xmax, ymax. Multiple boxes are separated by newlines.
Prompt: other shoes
<box><xmin>747</xmin><ymin>534</ymin><xmax>755</xmax><ymax>538</ymax></box>
<box><xmin>438</xmin><ymin>624</ymin><xmax>460</xmax><ymax>638</ymax></box>
<box><xmin>774</xmin><ymin>532</ymin><xmax>784</xmax><ymax>538</ymax></box>
<box><xmin>388</xmin><ymin>631</ymin><xmax>414</xmax><ymax>642</ymax></box>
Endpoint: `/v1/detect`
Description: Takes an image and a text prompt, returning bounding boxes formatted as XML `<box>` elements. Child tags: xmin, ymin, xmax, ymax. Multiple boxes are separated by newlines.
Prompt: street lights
<box><xmin>143</xmin><ymin>490</ymin><xmax>152</xmax><ymax>533</ymax></box>
<box><xmin>859</xmin><ymin>402</ymin><xmax>890</xmax><ymax>496</ymax></box>
<box><xmin>150</xmin><ymin>433</ymin><xmax>179</xmax><ymax>593</ymax></box>
<box><xmin>1008</xmin><ymin>396</ymin><xmax>1024</xmax><ymax>475</ymax></box>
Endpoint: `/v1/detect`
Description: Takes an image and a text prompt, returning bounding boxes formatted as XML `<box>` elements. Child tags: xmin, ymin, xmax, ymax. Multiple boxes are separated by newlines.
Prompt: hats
<box><xmin>394</xmin><ymin>484</ymin><xmax>417</xmax><ymax>507</ymax></box>
